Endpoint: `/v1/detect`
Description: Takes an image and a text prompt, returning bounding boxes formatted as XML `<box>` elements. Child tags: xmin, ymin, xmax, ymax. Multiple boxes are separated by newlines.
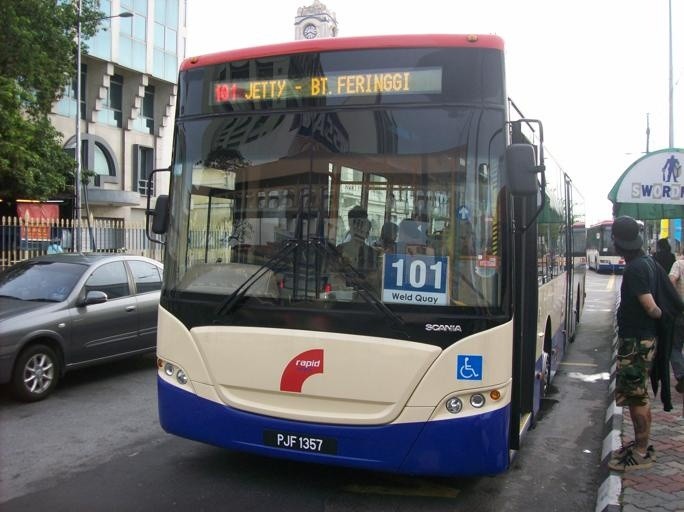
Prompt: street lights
<box><xmin>71</xmin><ymin>1</ymin><xmax>135</xmax><ymax>246</ymax></box>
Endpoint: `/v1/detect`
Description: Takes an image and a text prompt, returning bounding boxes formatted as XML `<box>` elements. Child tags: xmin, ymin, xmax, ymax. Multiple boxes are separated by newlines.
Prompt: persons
<box><xmin>651</xmin><ymin>237</ymin><xmax>677</xmax><ymax>275</ymax></box>
<box><xmin>606</xmin><ymin>214</ymin><xmax>663</xmax><ymax>471</ymax></box>
<box><xmin>47</xmin><ymin>237</ymin><xmax>64</xmax><ymax>255</ymax></box>
<box><xmin>662</xmin><ymin>155</ymin><xmax>679</xmax><ymax>182</ymax></box>
<box><xmin>316</xmin><ymin>205</ymin><xmax>498</xmax><ymax>291</ymax></box>
<box><xmin>668</xmin><ymin>246</ymin><xmax>683</xmax><ymax>394</ymax></box>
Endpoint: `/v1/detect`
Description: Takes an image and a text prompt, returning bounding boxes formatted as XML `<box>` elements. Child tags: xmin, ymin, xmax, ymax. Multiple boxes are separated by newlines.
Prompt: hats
<box><xmin>611</xmin><ymin>215</ymin><xmax>643</xmax><ymax>252</ymax></box>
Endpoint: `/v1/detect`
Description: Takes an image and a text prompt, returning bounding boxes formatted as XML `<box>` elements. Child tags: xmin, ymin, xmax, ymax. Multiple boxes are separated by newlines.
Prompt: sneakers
<box><xmin>607</xmin><ymin>443</ymin><xmax>657</xmax><ymax>471</ymax></box>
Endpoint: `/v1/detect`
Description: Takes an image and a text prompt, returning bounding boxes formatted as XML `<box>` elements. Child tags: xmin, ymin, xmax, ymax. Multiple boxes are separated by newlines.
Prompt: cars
<box><xmin>0</xmin><ymin>252</ymin><xmax>164</xmax><ymax>400</ymax></box>
<box><xmin>535</xmin><ymin>236</ymin><xmax>548</xmax><ymax>276</ymax></box>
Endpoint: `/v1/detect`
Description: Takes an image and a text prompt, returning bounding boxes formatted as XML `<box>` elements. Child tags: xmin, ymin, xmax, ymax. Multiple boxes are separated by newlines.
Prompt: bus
<box><xmin>586</xmin><ymin>218</ymin><xmax>644</xmax><ymax>276</ymax></box>
<box><xmin>555</xmin><ymin>221</ymin><xmax>585</xmax><ymax>273</ymax></box>
<box><xmin>144</xmin><ymin>33</ymin><xmax>590</xmax><ymax>483</ymax></box>
